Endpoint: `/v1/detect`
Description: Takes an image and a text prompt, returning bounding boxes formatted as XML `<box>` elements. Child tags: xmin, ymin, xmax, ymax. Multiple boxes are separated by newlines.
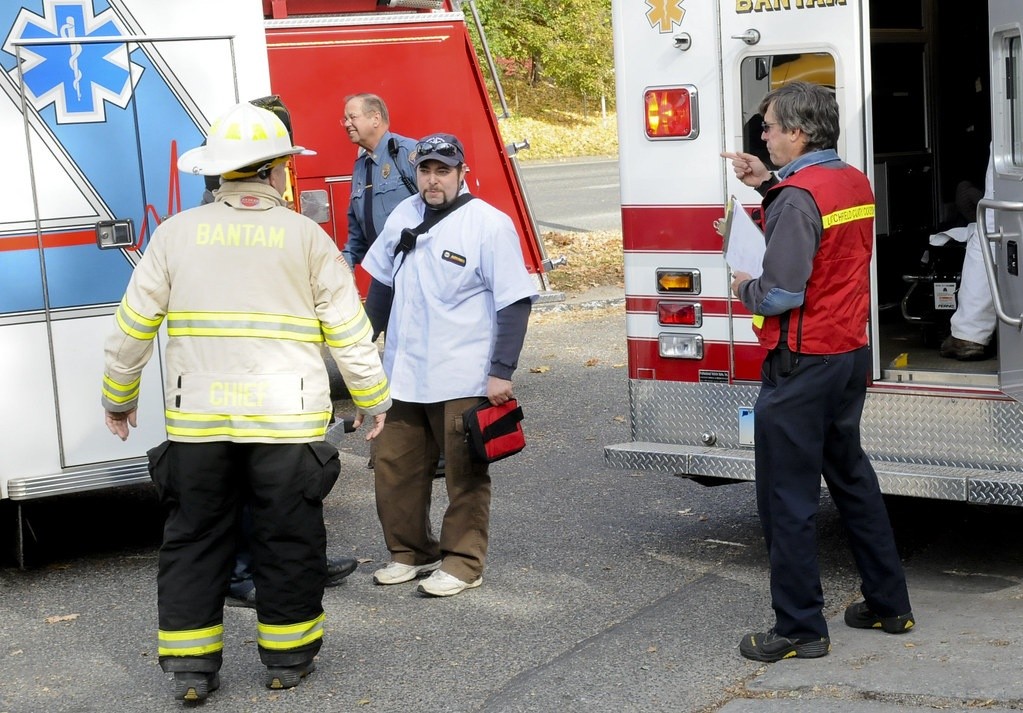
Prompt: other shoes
<box><xmin>174</xmin><ymin>669</ymin><xmax>220</xmax><ymax>701</ymax></box>
<box><xmin>940</xmin><ymin>334</ymin><xmax>984</xmax><ymax>361</ymax></box>
<box><xmin>433</xmin><ymin>458</ymin><xmax>448</xmax><ymax>475</ymax></box>
<box><xmin>266</xmin><ymin>663</ymin><xmax>315</xmax><ymax>688</ymax></box>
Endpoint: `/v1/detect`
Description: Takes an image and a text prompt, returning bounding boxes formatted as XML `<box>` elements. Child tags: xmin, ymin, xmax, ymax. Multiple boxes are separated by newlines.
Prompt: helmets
<box><xmin>177</xmin><ymin>102</ymin><xmax>304</xmax><ymax>176</ymax></box>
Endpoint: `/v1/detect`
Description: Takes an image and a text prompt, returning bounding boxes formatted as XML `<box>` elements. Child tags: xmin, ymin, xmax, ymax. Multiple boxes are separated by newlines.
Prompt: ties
<box><xmin>364</xmin><ymin>157</ymin><xmax>375</xmax><ymax>246</ymax></box>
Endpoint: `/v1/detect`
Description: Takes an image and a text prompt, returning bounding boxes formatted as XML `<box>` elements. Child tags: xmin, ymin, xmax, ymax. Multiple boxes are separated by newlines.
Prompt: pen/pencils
<box><xmin>730</xmin><ymin>273</ymin><xmax>736</xmax><ymax>279</ymax></box>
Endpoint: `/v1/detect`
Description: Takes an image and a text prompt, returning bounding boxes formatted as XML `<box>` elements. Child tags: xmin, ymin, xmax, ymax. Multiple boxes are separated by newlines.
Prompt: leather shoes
<box><xmin>324</xmin><ymin>559</ymin><xmax>358</xmax><ymax>583</ymax></box>
<box><xmin>226</xmin><ymin>585</ymin><xmax>260</xmax><ymax>610</ymax></box>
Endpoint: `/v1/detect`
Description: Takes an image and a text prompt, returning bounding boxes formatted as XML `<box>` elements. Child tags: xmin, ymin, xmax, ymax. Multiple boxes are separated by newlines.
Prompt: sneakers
<box><xmin>844</xmin><ymin>600</ymin><xmax>914</xmax><ymax>633</ymax></box>
<box><xmin>373</xmin><ymin>553</ymin><xmax>443</xmax><ymax>584</ymax></box>
<box><xmin>740</xmin><ymin>626</ymin><xmax>832</xmax><ymax>662</ymax></box>
<box><xmin>418</xmin><ymin>568</ymin><xmax>483</xmax><ymax>597</ymax></box>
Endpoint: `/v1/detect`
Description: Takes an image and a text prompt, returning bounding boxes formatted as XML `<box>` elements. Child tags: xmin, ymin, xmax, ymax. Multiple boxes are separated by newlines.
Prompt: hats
<box><xmin>413</xmin><ymin>135</ymin><xmax>463</xmax><ymax>169</ymax></box>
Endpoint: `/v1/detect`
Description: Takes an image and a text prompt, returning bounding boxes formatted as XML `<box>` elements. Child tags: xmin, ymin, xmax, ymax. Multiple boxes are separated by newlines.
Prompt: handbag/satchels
<box><xmin>462</xmin><ymin>396</ymin><xmax>526</xmax><ymax>465</ymax></box>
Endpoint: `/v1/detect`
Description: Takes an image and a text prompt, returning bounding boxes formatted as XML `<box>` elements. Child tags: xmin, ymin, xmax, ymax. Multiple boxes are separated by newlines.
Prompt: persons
<box><xmin>96</xmin><ymin>100</ymin><xmax>392</xmax><ymax>704</ymax></box>
<box><xmin>938</xmin><ymin>138</ymin><xmax>996</xmax><ymax>358</ymax></box>
<box><xmin>196</xmin><ymin>97</ymin><xmax>359</xmax><ymax>605</ymax></box>
<box><xmin>718</xmin><ymin>80</ymin><xmax>917</xmax><ymax>660</ymax></box>
<box><xmin>359</xmin><ymin>130</ymin><xmax>539</xmax><ymax>597</ymax></box>
<box><xmin>338</xmin><ymin>92</ymin><xmax>467</xmax><ymax>476</ymax></box>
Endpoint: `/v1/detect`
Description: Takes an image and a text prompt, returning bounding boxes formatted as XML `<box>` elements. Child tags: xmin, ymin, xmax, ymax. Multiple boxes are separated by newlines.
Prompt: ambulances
<box><xmin>2</xmin><ymin>2</ymin><xmax>556</xmax><ymax>504</ymax></box>
<box><xmin>612</xmin><ymin>0</ymin><xmax>1023</xmax><ymax>506</ymax></box>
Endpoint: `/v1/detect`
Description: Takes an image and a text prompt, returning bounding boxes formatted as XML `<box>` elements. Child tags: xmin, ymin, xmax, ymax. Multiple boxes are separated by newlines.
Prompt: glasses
<box><xmin>761</xmin><ymin>120</ymin><xmax>785</xmax><ymax>134</ymax></box>
<box><xmin>340</xmin><ymin>109</ymin><xmax>377</xmax><ymax>127</ymax></box>
<box><xmin>415</xmin><ymin>142</ymin><xmax>464</xmax><ymax>157</ymax></box>
